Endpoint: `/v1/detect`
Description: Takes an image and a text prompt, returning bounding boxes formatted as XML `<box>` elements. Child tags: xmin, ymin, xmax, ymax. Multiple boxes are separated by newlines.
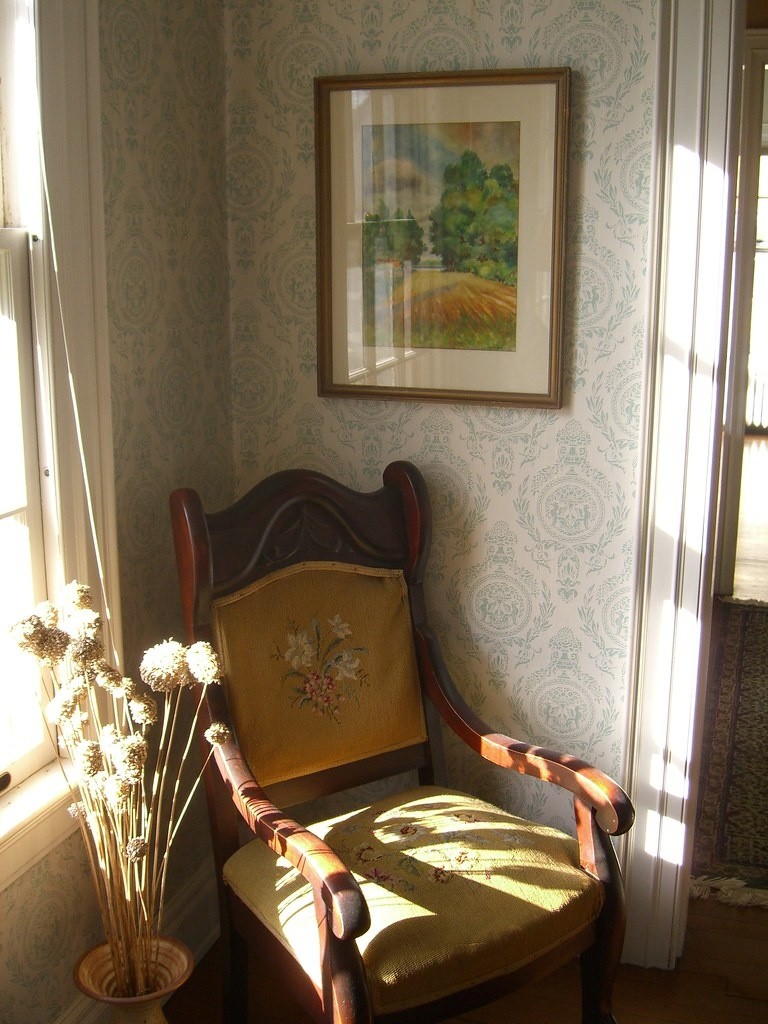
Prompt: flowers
<box><xmin>8</xmin><ymin>582</ymin><xmax>231</xmax><ymax>992</ymax></box>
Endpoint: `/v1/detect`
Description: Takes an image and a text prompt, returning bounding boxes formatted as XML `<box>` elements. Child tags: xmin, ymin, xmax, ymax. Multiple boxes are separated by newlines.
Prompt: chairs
<box><xmin>168</xmin><ymin>462</ymin><xmax>638</xmax><ymax>1024</ymax></box>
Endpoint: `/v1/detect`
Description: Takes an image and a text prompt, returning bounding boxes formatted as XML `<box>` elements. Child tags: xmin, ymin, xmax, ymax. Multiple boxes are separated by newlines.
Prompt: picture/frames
<box><xmin>311</xmin><ymin>65</ymin><xmax>572</xmax><ymax>408</ymax></box>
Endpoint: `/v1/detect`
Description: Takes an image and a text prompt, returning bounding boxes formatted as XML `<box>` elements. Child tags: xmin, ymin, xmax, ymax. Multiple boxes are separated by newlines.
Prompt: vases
<box><xmin>75</xmin><ymin>940</ymin><xmax>197</xmax><ymax>1023</ymax></box>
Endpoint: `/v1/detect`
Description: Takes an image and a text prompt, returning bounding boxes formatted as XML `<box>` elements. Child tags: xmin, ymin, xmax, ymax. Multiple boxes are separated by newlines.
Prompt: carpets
<box><xmin>689</xmin><ymin>593</ymin><xmax>768</xmax><ymax>909</ymax></box>
